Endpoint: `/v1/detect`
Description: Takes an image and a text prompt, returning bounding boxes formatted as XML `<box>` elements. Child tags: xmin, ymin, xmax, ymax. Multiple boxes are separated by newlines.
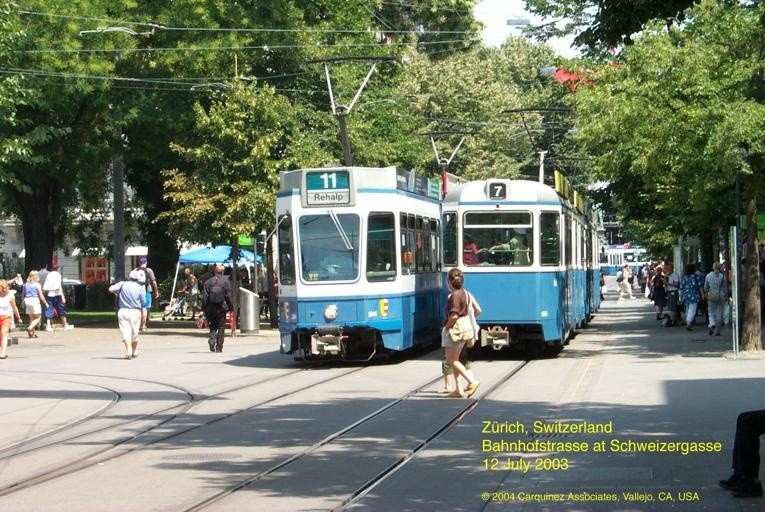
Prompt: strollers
<box><xmin>162</xmin><ymin>292</ymin><xmax>187</xmax><ymax>321</ymax></box>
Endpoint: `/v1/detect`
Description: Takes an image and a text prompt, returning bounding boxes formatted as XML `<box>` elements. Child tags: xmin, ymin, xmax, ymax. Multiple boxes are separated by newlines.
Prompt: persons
<box><xmin>616</xmin><ymin>263</ymin><xmax>734</xmax><ymax>336</ymax></box>
<box><xmin>599</xmin><ymin>268</ymin><xmax>608</xmax><ymax>300</ymax></box>
<box><xmin>229</xmin><ymin>267</ymin><xmax>279</xmax><ymax>328</ymax></box>
<box><xmin>462</xmin><ymin>228</ymin><xmax>530</xmax><ymax>267</ymax></box>
<box><xmin>320</xmin><ymin>240</ymin><xmax>357</xmax><ymax>270</ymax></box>
<box><xmin>718</xmin><ymin>410</ymin><xmax>765</xmax><ymax>498</ymax></box>
<box><xmin>444</xmin><ymin>268</ymin><xmax>482</xmax><ymax>399</ymax></box>
<box><xmin>108</xmin><ymin>269</ymin><xmax>146</xmax><ymax>360</ymax></box>
<box><xmin>137</xmin><ymin>256</ymin><xmax>159</xmax><ymax>326</ymax></box>
<box><xmin>42</xmin><ymin>263</ymin><xmax>75</xmax><ymax>331</ymax></box>
<box><xmin>177</xmin><ymin>267</ymin><xmax>203</xmax><ymax>321</ymax></box>
<box><xmin>37</xmin><ymin>261</ymin><xmax>50</xmax><ymax>332</ymax></box>
<box><xmin>20</xmin><ymin>270</ymin><xmax>50</xmax><ymax>338</ymax></box>
<box><xmin>202</xmin><ymin>265</ymin><xmax>234</xmax><ymax>353</ymax></box>
<box><xmin>0</xmin><ymin>279</ymin><xmax>23</xmax><ymax>360</ymax></box>
<box><xmin>440</xmin><ymin>307</ymin><xmax>470</xmax><ymax>394</ymax></box>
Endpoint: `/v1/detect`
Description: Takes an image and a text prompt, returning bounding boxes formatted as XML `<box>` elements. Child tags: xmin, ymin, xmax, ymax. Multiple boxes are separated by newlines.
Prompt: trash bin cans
<box><xmin>239</xmin><ymin>287</ymin><xmax>261</xmax><ymax>334</ymax></box>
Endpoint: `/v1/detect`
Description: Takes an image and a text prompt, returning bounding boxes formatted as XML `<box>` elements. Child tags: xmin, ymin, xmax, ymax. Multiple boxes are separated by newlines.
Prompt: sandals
<box><xmin>25</xmin><ymin>326</ymin><xmax>39</xmax><ymax>339</ymax></box>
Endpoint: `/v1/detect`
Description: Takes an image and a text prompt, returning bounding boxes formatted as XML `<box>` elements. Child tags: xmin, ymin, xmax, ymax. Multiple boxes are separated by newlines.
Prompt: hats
<box><xmin>128</xmin><ymin>269</ymin><xmax>141</xmax><ymax>279</ymax></box>
<box><xmin>138</xmin><ymin>256</ymin><xmax>148</xmax><ymax>264</ymax></box>
<box><xmin>512</xmin><ymin>228</ymin><xmax>528</xmax><ymax>235</ymax></box>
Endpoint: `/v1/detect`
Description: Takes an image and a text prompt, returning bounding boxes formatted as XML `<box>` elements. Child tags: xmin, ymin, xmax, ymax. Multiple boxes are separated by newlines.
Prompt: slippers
<box><xmin>0</xmin><ymin>352</ymin><xmax>8</xmax><ymax>360</ymax></box>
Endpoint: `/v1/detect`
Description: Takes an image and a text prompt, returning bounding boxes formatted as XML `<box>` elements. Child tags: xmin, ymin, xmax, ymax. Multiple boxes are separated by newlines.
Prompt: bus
<box><xmin>601</xmin><ymin>245</ymin><xmax>651</xmax><ymax>275</ymax></box>
<box><xmin>439</xmin><ymin>134</ymin><xmax>603</xmax><ymax>352</ymax></box>
<box><xmin>276</xmin><ymin>63</ymin><xmax>439</xmax><ymax>365</ymax></box>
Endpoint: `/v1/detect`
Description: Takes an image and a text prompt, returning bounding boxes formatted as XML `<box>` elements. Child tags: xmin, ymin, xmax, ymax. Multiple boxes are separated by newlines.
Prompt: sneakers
<box><xmin>731</xmin><ymin>480</ymin><xmax>763</xmax><ymax>497</ymax></box>
<box><xmin>719</xmin><ymin>475</ymin><xmax>740</xmax><ymax>489</ymax></box>
<box><xmin>442</xmin><ymin>385</ymin><xmax>454</xmax><ymax>393</ymax></box>
<box><xmin>715</xmin><ymin>333</ymin><xmax>722</xmax><ymax>337</ymax></box>
<box><xmin>45</xmin><ymin>325</ymin><xmax>53</xmax><ymax>332</ymax></box>
<box><xmin>123</xmin><ymin>351</ymin><xmax>133</xmax><ymax>360</ymax></box>
<box><xmin>131</xmin><ymin>350</ymin><xmax>141</xmax><ymax>358</ymax></box>
<box><xmin>465</xmin><ymin>381</ymin><xmax>480</xmax><ymax>398</ymax></box>
<box><xmin>216</xmin><ymin>343</ymin><xmax>224</xmax><ymax>353</ymax></box>
<box><xmin>63</xmin><ymin>324</ymin><xmax>74</xmax><ymax>331</ymax></box>
<box><xmin>709</xmin><ymin>325</ymin><xmax>716</xmax><ymax>335</ymax></box>
<box><xmin>445</xmin><ymin>389</ymin><xmax>464</xmax><ymax>398</ymax></box>
<box><xmin>208</xmin><ymin>342</ymin><xmax>216</xmax><ymax>353</ymax></box>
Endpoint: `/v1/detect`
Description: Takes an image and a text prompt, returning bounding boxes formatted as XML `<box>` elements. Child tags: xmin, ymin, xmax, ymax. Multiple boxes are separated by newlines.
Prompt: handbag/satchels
<box><xmin>706</xmin><ymin>291</ymin><xmax>721</xmax><ymax>301</ymax></box>
<box><xmin>466</xmin><ymin>307</ymin><xmax>481</xmax><ymax>349</ymax></box>
<box><xmin>723</xmin><ymin>303</ymin><xmax>731</xmax><ymax>325</ymax></box>
<box><xmin>449</xmin><ymin>314</ymin><xmax>474</xmax><ymax>343</ymax></box>
<box><xmin>114</xmin><ymin>294</ymin><xmax>119</xmax><ymax>311</ymax></box>
<box><xmin>19</xmin><ymin>299</ymin><xmax>25</xmax><ymax>309</ymax></box>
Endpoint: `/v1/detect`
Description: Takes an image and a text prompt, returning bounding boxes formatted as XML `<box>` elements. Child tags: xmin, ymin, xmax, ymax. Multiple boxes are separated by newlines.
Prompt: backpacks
<box><xmin>208</xmin><ymin>286</ymin><xmax>225</xmax><ymax>305</ymax></box>
